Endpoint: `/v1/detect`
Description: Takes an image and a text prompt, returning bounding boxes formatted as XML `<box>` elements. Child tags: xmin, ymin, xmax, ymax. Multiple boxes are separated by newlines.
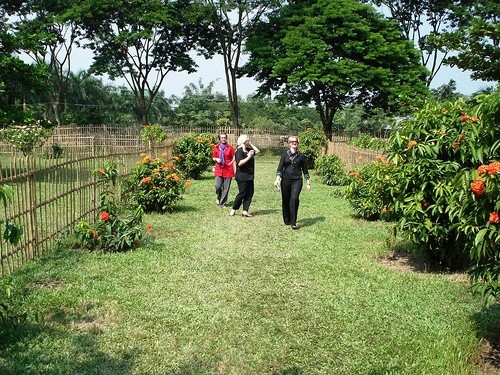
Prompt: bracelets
<box><xmin>214</xmin><ymin>158</ymin><xmax>216</xmax><ymax>161</ymax></box>
<box><xmin>306</xmin><ymin>184</ymin><xmax>310</xmax><ymax>185</ymax></box>
<box><xmin>275</xmin><ymin>177</ymin><xmax>280</xmax><ymax>180</ymax></box>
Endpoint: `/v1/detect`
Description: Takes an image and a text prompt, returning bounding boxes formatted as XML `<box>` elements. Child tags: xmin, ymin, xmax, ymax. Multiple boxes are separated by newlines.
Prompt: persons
<box><xmin>274</xmin><ymin>136</ymin><xmax>311</xmax><ymax>230</ymax></box>
<box><xmin>229</xmin><ymin>135</ymin><xmax>260</xmax><ymax>217</ymax></box>
<box><xmin>212</xmin><ymin>133</ymin><xmax>235</xmax><ymax>209</ymax></box>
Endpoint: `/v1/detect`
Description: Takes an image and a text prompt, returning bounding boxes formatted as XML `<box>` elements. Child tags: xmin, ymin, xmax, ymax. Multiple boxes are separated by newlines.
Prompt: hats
<box><xmin>237</xmin><ymin>134</ymin><xmax>247</xmax><ymax>145</ymax></box>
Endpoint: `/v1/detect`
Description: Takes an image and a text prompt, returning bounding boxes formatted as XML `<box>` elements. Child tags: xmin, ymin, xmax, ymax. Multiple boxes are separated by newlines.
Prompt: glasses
<box><xmin>288</xmin><ymin>141</ymin><xmax>298</xmax><ymax>145</ymax></box>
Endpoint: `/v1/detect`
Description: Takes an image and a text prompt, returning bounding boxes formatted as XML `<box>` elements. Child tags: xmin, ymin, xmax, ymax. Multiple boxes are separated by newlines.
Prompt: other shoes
<box><xmin>291</xmin><ymin>224</ymin><xmax>297</xmax><ymax>230</ymax></box>
<box><xmin>241</xmin><ymin>211</ymin><xmax>252</xmax><ymax>217</ymax></box>
<box><xmin>230</xmin><ymin>210</ymin><xmax>236</xmax><ymax>216</ymax></box>
